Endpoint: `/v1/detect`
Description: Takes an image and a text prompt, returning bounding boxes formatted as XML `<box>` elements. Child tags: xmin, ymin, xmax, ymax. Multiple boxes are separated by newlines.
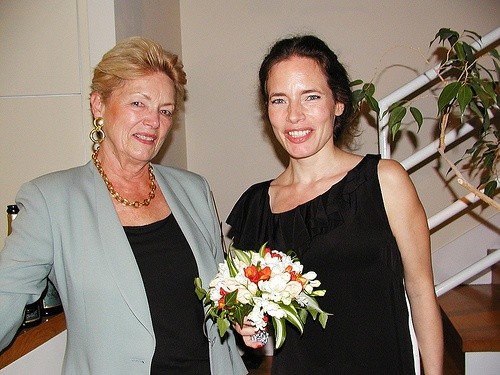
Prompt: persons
<box><xmin>1</xmin><ymin>36</ymin><xmax>252</xmax><ymax>375</ymax></box>
<box><xmin>225</xmin><ymin>34</ymin><xmax>445</xmax><ymax>375</ymax></box>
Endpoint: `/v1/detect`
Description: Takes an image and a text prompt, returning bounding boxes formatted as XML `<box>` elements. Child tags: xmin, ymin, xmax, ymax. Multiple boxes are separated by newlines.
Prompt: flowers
<box><xmin>194</xmin><ymin>236</ymin><xmax>334</xmax><ymax>346</ymax></box>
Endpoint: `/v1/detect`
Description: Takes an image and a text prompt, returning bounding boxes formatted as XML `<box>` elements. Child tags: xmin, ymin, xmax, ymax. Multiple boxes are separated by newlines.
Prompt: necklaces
<box><xmin>90</xmin><ymin>147</ymin><xmax>156</xmax><ymax>208</ymax></box>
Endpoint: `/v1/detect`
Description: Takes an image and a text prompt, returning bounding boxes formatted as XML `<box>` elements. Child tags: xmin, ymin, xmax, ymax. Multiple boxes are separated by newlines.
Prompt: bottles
<box><xmin>7</xmin><ymin>204</ymin><xmax>41</xmax><ymax>327</ymax></box>
<box><xmin>40</xmin><ymin>276</ymin><xmax>64</xmax><ymax>316</ymax></box>
